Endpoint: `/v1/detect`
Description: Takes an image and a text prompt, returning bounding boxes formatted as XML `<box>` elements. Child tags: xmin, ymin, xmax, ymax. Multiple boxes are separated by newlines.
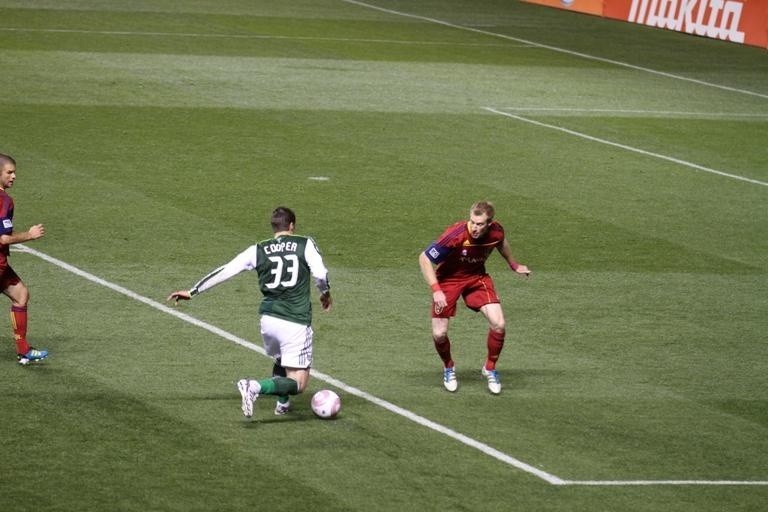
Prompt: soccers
<box><xmin>310</xmin><ymin>389</ymin><xmax>341</xmax><ymax>419</ymax></box>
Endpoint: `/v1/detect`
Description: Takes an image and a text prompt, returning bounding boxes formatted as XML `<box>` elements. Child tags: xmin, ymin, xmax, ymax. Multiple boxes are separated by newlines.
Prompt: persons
<box><xmin>166</xmin><ymin>206</ymin><xmax>333</xmax><ymax>417</ymax></box>
<box><xmin>418</xmin><ymin>201</ymin><xmax>532</xmax><ymax>395</ymax></box>
<box><xmin>0</xmin><ymin>153</ymin><xmax>50</xmax><ymax>366</ymax></box>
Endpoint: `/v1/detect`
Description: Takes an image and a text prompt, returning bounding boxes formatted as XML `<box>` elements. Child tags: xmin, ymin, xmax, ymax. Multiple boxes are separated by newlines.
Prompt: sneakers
<box><xmin>237</xmin><ymin>378</ymin><xmax>259</xmax><ymax>418</ymax></box>
<box><xmin>482</xmin><ymin>365</ymin><xmax>502</xmax><ymax>394</ymax></box>
<box><xmin>17</xmin><ymin>347</ymin><xmax>48</xmax><ymax>365</ymax></box>
<box><xmin>274</xmin><ymin>404</ymin><xmax>295</xmax><ymax>415</ymax></box>
<box><xmin>444</xmin><ymin>362</ymin><xmax>458</xmax><ymax>392</ymax></box>
